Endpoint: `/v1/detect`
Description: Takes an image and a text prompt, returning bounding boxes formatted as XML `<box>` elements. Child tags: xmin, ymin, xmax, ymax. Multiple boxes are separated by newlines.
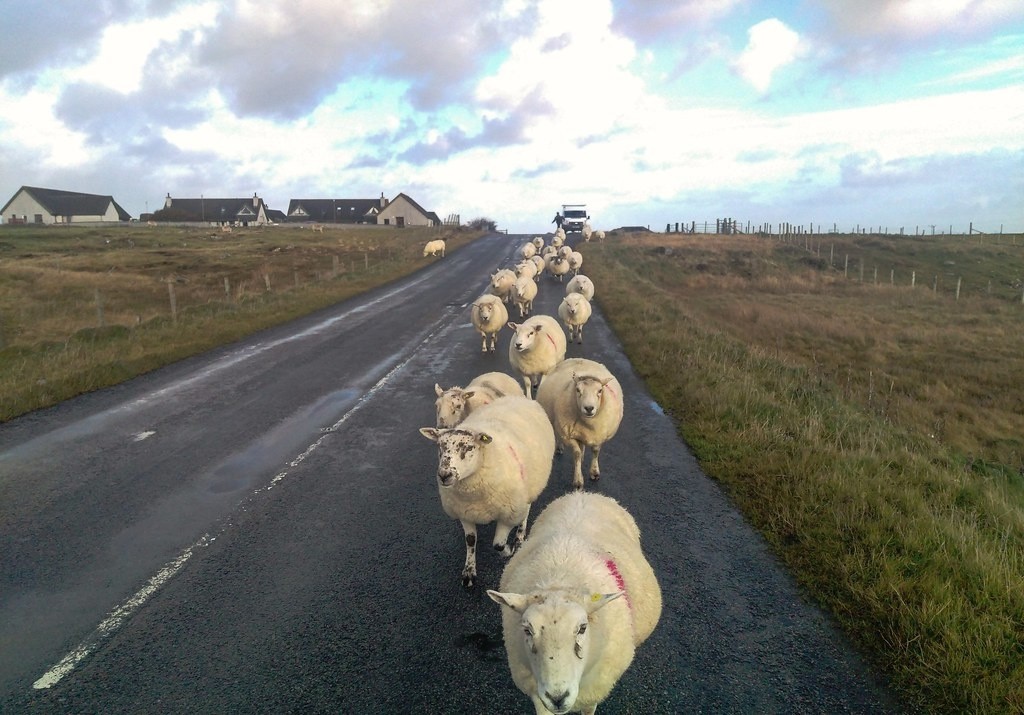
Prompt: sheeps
<box><xmin>418</xmin><ymin>356</ymin><xmax>624</xmax><ymax>587</ymax></box>
<box><xmin>472</xmin><ymin>227</ymin><xmax>595</xmax><ymax>400</ymax></box>
<box><xmin>581</xmin><ymin>223</ymin><xmax>605</xmax><ymax>245</ymax></box>
<box><xmin>484</xmin><ymin>491</ymin><xmax>665</xmax><ymax>715</ymax></box>
<box><xmin>423</xmin><ymin>240</ymin><xmax>446</xmax><ymax>259</ymax></box>
